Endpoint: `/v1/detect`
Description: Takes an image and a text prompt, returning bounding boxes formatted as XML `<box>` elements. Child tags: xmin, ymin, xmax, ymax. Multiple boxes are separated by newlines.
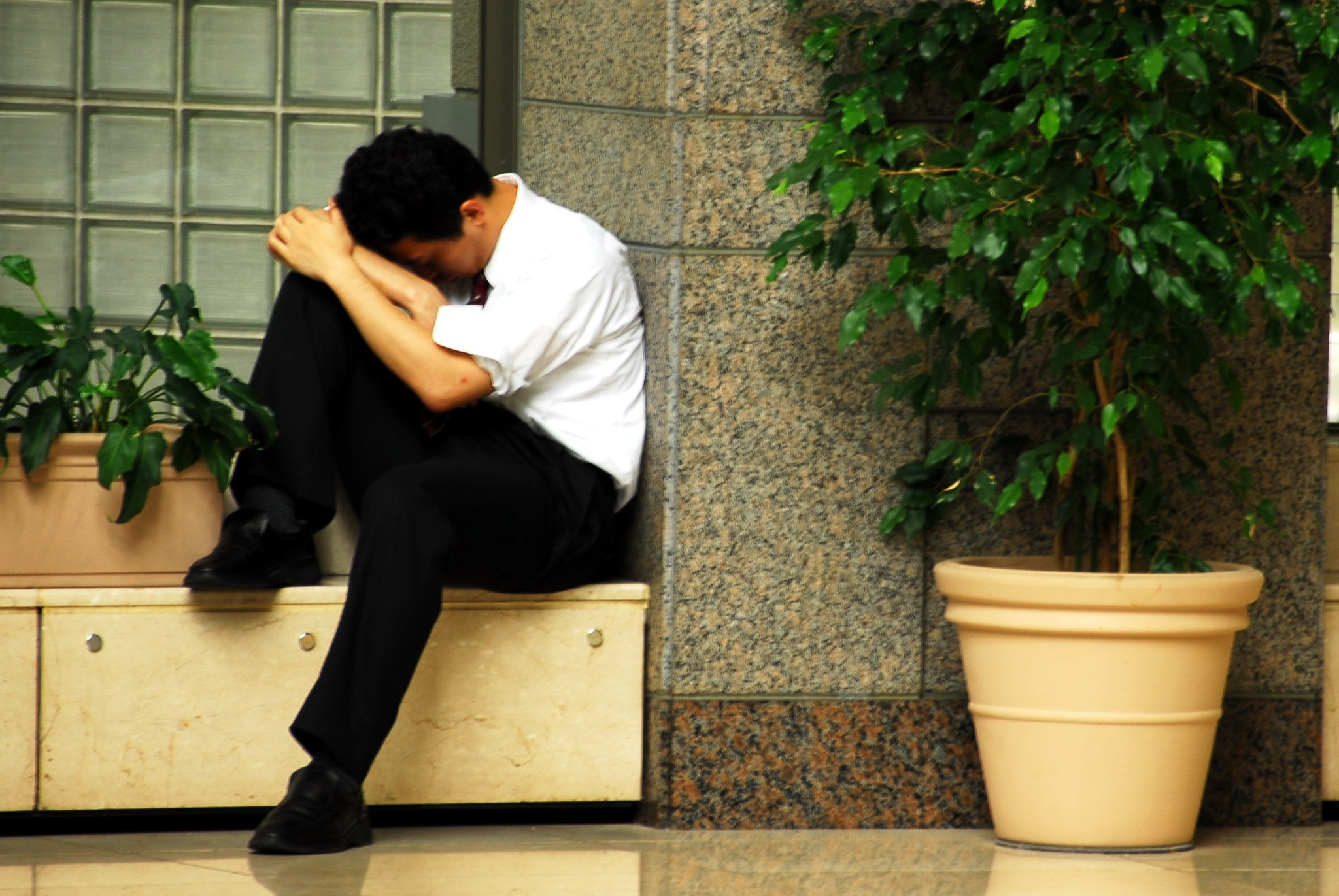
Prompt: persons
<box><xmin>183</xmin><ymin>120</ymin><xmax>646</xmax><ymax>855</ymax></box>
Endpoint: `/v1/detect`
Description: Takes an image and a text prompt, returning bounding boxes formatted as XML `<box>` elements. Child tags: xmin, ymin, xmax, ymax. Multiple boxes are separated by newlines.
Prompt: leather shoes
<box><xmin>182</xmin><ymin>522</ymin><xmax>323</xmax><ymax>589</ymax></box>
<box><xmin>246</xmin><ymin>767</ymin><xmax>374</xmax><ymax>854</ymax></box>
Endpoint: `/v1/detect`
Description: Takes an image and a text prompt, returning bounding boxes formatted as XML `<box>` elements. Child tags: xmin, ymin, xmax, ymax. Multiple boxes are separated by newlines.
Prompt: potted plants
<box><xmin>761</xmin><ymin>0</ymin><xmax>1339</xmax><ymax>853</ymax></box>
<box><xmin>0</xmin><ymin>254</ymin><xmax>275</xmax><ymax>587</ymax></box>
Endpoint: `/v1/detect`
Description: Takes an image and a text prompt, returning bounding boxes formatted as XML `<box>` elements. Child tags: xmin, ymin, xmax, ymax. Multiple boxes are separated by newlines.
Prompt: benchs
<box><xmin>0</xmin><ymin>583</ymin><xmax>649</xmax><ymax>811</ymax></box>
